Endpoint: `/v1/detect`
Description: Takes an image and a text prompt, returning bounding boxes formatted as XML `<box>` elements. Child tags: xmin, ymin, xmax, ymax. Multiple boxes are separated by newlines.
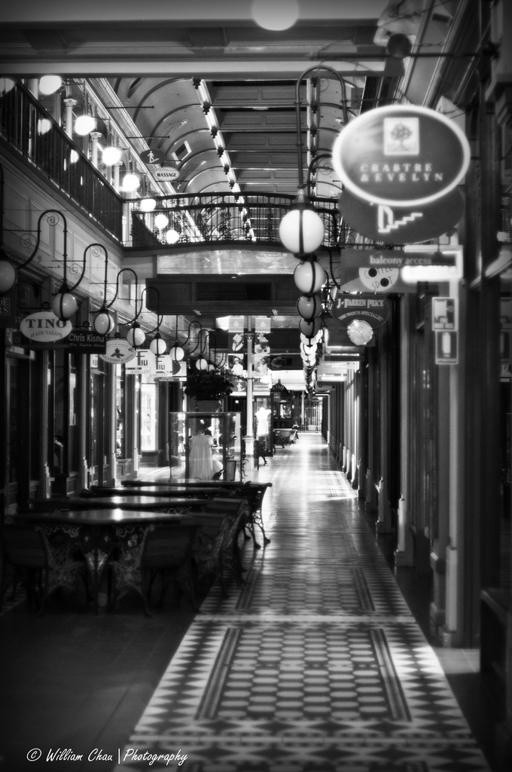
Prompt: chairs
<box><xmin>2</xmin><ymin>474</ymin><xmax>274</xmax><ymax>617</ymax></box>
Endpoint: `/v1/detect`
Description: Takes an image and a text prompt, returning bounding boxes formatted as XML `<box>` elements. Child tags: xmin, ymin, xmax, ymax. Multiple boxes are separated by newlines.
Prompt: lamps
<box><xmin>181</xmin><ymin>320</ymin><xmax>249</xmax><ymax>382</ymax></box>
<box><xmin>146</xmin><ymin>307</ymin><xmax>185</xmax><ymax>363</ymax></box>
<box><xmin>37</xmin><ymin>76</ymin><xmax>184</xmax><ymax>245</ymax></box>
<box><xmin>279</xmin><ymin>67</ymin><xmax>348</xmax><ymax>418</ymax></box>
<box><xmin>124</xmin><ymin>287</ymin><xmax>168</xmax><ymax>358</ymax></box>
<box><xmin>14</xmin><ymin>208</ymin><xmax>80</xmax><ymax>322</ymax></box>
<box><xmin>90</xmin><ymin>267</ymin><xmax>146</xmax><ymax>351</ymax></box>
<box><xmin>54</xmin><ymin>243</ymin><xmax>115</xmax><ymax>335</ymax></box>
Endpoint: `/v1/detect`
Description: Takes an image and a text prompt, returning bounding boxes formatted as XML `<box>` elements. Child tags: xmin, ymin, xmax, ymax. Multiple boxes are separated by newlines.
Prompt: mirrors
<box><xmin>253</xmin><ymin>394</ymin><xmax>275</xmax><ymax>457</ymax></box>
<box><xmin>185</xmin><ymin>411</ymin><xmax>241</xmax><ymax>481</ymax></box>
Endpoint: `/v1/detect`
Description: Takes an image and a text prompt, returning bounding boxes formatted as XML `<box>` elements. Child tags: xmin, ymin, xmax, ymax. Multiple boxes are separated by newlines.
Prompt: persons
<box><xmin>212</xmin><ymin>457</ymin><xmax>224</xmax><ymax>480</ymax></box>
<box><xmin>188</xmin><ymin>427</ymin><xmax>213</xmax><ymax>480</ymax></box>
